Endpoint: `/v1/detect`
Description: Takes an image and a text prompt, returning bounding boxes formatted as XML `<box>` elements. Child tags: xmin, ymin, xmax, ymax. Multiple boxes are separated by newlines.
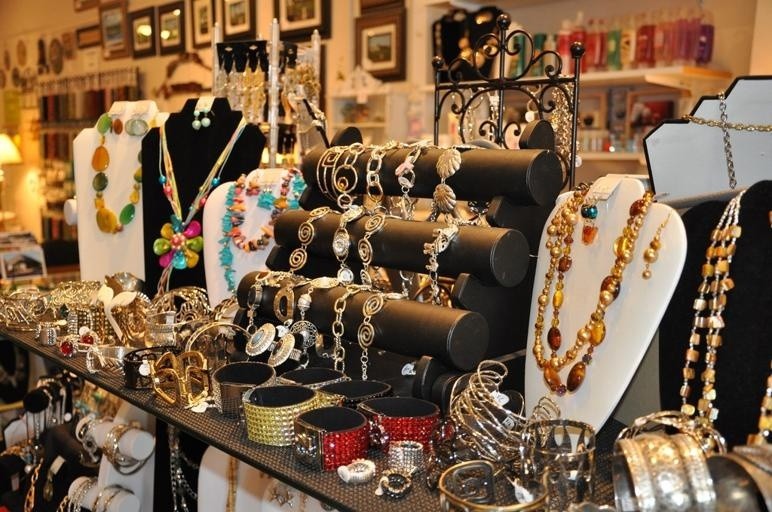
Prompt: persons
<box><xmin>282</xmin><ymin>64</ymin><xmax>326</xmax><ymax>162</ymax></box>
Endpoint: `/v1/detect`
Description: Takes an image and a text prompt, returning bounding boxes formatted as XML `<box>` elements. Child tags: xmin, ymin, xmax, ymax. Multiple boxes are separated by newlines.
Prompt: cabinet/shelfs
<box><xmin>330</xmin><ymin>69</ymin><xmax>409</xmax><ymax>142</ymax></box>
<box><xmin>2</xmin><ymin>288</ymin><xmax>772</xmax><ymax>512</ymax></box>
<box><xmin>500</xmin><ymin>63</ymin><xmax>730</xmax><ymax>178</ymax></box>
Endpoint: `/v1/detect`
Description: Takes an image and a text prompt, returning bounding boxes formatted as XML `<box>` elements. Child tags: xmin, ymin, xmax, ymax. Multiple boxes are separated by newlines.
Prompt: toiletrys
<box><xmin>699</xmin><ymin>11</ymin><xmax>716</xmax><ymax>66</ymax></box>
<box><xmin>584</xmin><ymin>19</ymin><xmax>600</xmax><ymax>72</ymax></box>
<box><xmin>667</xmin><ymin>8</ymin><xmax>680</xmax><ymax>64</ymax></box>
<box><xmin>41</xmin><ymin>207</ymin><xmax>77</xmax><ymax>244</ymax></box>
<box><xmin>687</xmin><ymin>3</ymin><xmax>701</xmax><ymax>67</ymax></box>
<box><xmin>598</xmin><ymin>18</ymin><xmax>606</xmax><ymax>70</ymax></box>
<box><xmin>659</xmin><ymin>9</ymin><xmax>675</xmax><ymax>65</ymax></box>
<box><xmin>570</xmin><ymin>10</ymin><xmax>587</xmax><ymax>73</ymax></box>
<box><xmin>532</xmin><ymin>32</ymin><xmax>545</xmax><ymax>75</ymax></box>
<box><xmin>620</xmin><ymin>13</ymin><xmax>638</xmax><ymax>70</ymax></box>
<box><xmin>631</xmin><ymin>103</ymin><xmax>659</xmax><ymax>126</ymax></box>
<box><xmin>509</xmin><ymin>26</ymin><xmax>523</xmax><ymax>79</ymax></box>
<box><xmin>37</xmin><ymin>68</ymin><xmax>139</xmax><ymax>123</ymax></box>
<box><xmin>543</xmin><ymin>32</ymin><xmax>558</xmax><ymax>76</ymax></box>
<box><xmin>557</xmin><ymin>19</ymin><xmax>571</xmax><ymax>75</ymax></box>
<box><xmin>676</xmin><ymin>5</ymin><xmax>688</xmax><ymax>67</ymax></box>
<box><xmin>635</xmin><ymin>12</ymin><xmax>658</xmax><ymax>69</ymax></box>
<box><xmin>524</xmin><ymin>34</ymin><xmax>533</xmax><ymax>76</ymax></box>
<box><xmin>651</xmin><ymin>9</ymin><xmax>666</xmax><ymax>66</ymax></box>
<box><xmin>43</xmin><ymin>130</ymin><xmax>76</xmax><ymax>159</ymax></box>
<box><xmin>583</xmin><ymin>132</ymin><xmax>644</xmax><ymax>153</ymax></box>
<box><xmin>606</xmin><ymin>14</ymin><xmax>622</xmax><ymax>70</ymax></box>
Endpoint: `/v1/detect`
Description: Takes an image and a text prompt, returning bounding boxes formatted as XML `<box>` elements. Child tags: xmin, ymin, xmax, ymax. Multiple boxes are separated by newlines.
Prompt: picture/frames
<box><xmin>128</xmin><ymin>7</ymin><xmax>156</xmax><ymax>58</ymax></box>
<box><xmin>221</xmin><ymin>0</ymin><xmax>256</xmax><ymax>39</ymax></box>
<box><xmin>274</xmin><ymin>0</ymin><xmax>331</xmax><ymax>40</ymax></box>
<box><xmin>76</xmin><ymin>25</ymin><xmax>101</xmax><ymax>49</ymax></box>
<box><xmin>192</xmin><ymin>0</ymin><xmax>214</xmax><ymax>49</ymax></box>
<box><xmin>353</xmin><ymin>13</ymin><xmax>408</xmax><ymax>79</ymax></box>
<box><xmin>99</xmin><ymin>0</ymin><xmax>129</xmax><ymax>62</ymax></box>
<box><xmin>159</xmin><ymin>1</ymin><xmax>186</xmax><ymax>55</ymax></box>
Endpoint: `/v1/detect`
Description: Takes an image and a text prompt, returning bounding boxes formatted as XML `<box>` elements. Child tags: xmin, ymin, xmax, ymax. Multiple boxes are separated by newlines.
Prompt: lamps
<box><xmin>0</xmin><ymin>133</ymin><xmax>24</xmax><ymax>241</ymax></box>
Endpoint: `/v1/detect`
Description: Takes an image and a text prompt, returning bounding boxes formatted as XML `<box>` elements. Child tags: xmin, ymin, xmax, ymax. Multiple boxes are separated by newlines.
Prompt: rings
<box><xmin>335</xmin><ymin>458</ymin><xmax>376</xmax><ymax>483</ymax></box>
<box><xmin>391</xmin><ymin>442</ymin><xmax>426</xmax><ymax>476</ymax></box>
<box><xmin>375</xmin><ymin>471</ymin><xmax>413</xmax><ymax>498</ymax></box>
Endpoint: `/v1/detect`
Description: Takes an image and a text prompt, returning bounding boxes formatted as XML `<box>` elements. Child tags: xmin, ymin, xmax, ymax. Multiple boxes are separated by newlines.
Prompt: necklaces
<box><xmin>676</xmin><ymin>193</ymin><xmax>772</xmax><ymax>451</ymax></box>
<box><xmin>93</xmin><ymin>114</ymin><xmax>158</xmax><ymax>234</ymax></box>
<box><xmin>435</xmin><ymin>20</ymin><xmax>460</xmax><ymax>71</ymax></box>
<box><xmin>153</xmin><ymin>116</ymin><xmax>248</xmax><ymax>296</ymax></box>
<box><xmin>350</xmin><ymin>70</ymin><xmax>368</xmax><ymax>91</ymax></box>
<box><xmin>217</xmin><ymin>174</ymin><xmax>306</xmax><ymax>294</ymax></box>
<box><xmin>532</xmin><ymin>181</ymin><xmax>651</xmax><ymax>397</ymax></box>
<box><xmin>225</xmin><ymin>456</ymin><xmax>242</xmax><ymax>512</ymax></box>
<box><xmin>459</xmin><ymin>25</ymin><xmax>500</xmax><ymax>68</ymax></box>
<box><xmin>231</xmin><ymin>169</ymin><xmax>296</xmax><ymax>251</ymax></box>
<box><xmin>168</xmin><ymin>424</ymin><xmax>197</xmax><ymax>512</ymax></box>
<box><xmin>682</xmin><ymin>91</ymin><xmax>771</xmax><ymax>191</ymax></box>
<box><xmin>12</xmin><ymin>373</ymin><xmax>68</xmax><ymax>439</ymax></box>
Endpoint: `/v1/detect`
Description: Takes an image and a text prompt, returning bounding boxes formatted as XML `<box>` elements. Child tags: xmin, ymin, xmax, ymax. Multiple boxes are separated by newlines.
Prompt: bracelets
<box><xmin>87</xmin><ymin>346</ymin><xmax>139</xmax><ymax>376</ymax></box>
<box><xmin>359</xmin><ymin>215</ymin><xmax>414</xmax><ymax>299</ymax></box>
<box><xmin>365</xmin><ymin>141</ymin><xmax>409</xmax><ymax>216</ymax></box>
<box><xmin>276</xmin><ymin>276</ymin><xmax>311</xmax><ymax>322</ymax></box>
<box><xmin>292</xmin><ymin>406</ymin><xmax>369</xmax><ymax>471</ymax></box>
<box><xmin>124</xmin><ymin>346</ymin><xmax>182</xmax><ymax>390</ymax></box>
<box><xmin>104</xmin><ymin>421</ymin><xmax>157</xmax><ymax>476</ymax></box>
<box><xmin>150</xmin><ymin>285</ymin><xmax>211</xmax><ymax>322</ymax></box>
<box><xmin>33</xmin><ymin>320</ymin><xmax>87</xmax><ymax>345</ymax></box>
<box><xmin>66</xmin><ymin>476</ymin><xmax>100</xmax><ymax>511</ymax></box>
<box><xmin>1</xmin><ymin>291</ymin><xmax>43</xmax><ymax>331</ymax></box>
<box><xmin>394</xmin><ymin>139</ymin><xmax>438</xmax><ymax>222</ymax></box>
<box><xmin>317</xmin><ymin>145</ymin><xmax>351</xmax><ymax>204</ymax></box>
<box><xmin>334</xmin><ymin>142</ymin><xmax>376</xmax><ymax>209</ymax></box>
<box><xmin>57</xmin><ymin>326</ymin><xmax>115</xmax><ymax>358</ymax></box>
<box><xmin>320</xmin><ymin>380</ymin><xmax>391</xmax><ymax>411</ymax></box>
<box><xmin>522</xmin><ymin>420</ymin><xmax>596</xmax><ymax>512</ymax></box>
<box><xmin>670</xmin><ymin>430</ymin><xmax>718</xmax><ymax>512</ymax></box>
<box><xmin>93</xmin><ymin>484</ymin><xmax>135</xmax><ymax>512</ymax></box>
<box><xmin>145</xmin><ymin>311</ymin><xmax>188</xmax><ymax>349</ymax></box>
<box><xmin>185</xmin><ymin>321</ymin><xmax>253</xmax><ymax>370</ymax></box>
<box><xmin>81</xmin><ymin>416</ymin><xmax>114</xmax><ymax>464</ymax></box>
<box><xmin>435</xmin><ymin>143</ymin><xmax>496</xmax><ymax>228</ymax></box>
<box><xmin>152</xmin><ymin>351</ymin><xmax>209</xmax><ymax>407</ymax></box>
<box><xmin>211</xmin><ymin>296</ymin><xmax>241</xmax><ymax>335</ymax></box>
<box><xmin>359</xmin><ymin>396</ymin><xmax>438</xmax><ymax>453</ymax></box>
<box><xmin>239</xmin><ymin>386</ymin><xmax>321</xmax><ymax>446</ymax></box>
<box><xmin>613</xmin><ymin>437</ymin><xmax>659</xmax><ymax>511</ymax></box>
<box><xmin>276</xmin><ymin>367</ymin><xmax>347</xmax><ymax>391</ymax></box>
<box><xmin>293</xmin><ymin>275</ymin><xmax>348</xmax><ymax>359</ymax></box>
<box><xmin>633</xmin><ymin>428</ymin><xmax>693</xmax><ymax>512</ymax></box>
<box><xmin>244</xmin><ymin>270</ymin><xmax>291</xmax><ymax>334</ymax></box>
<box><xmin>437</xmin><ymin>460</ymin><xmax>551</xmax><ymax>512</ymax></box>
<box><xmin>287</xmin><ymin>207</ymin><xmax>341</xmax><ymax>275</ymax></box>
<box><xmin>709</xmin><ymin>444</ymin><xmax>772</xmax><ymax>511</ymax></box>
<box><xmin>614</xmin><ymin>410</ymin><xmax>726</xmax><ymax>460</ymax></box>
<box><xmin>359</xmin><ymin>292</ymin><xmax>402</xmax><ymax>380</ymax></box>
<box><xmin>450</xmin><ymin>361</ymin><xmax>562</xmax><ymax>467</ymax></box>
<box><xmin>49</xmin><ymin>274</ymin><xmax>149</xmax><ymax>321</ymax></box>
<box><xmin>414</xmin><ymin>222</ymin><xmax>470</xmax><ymax>311</ymax></box>
<box><xmin>211</xmin><ymin>362</ymin><xmax>276</xmax><ymax>417</ymax></box>
<box><xmin>333</xmin><ymin>205</ymin><xmax>368</xmax><ymax>285</ymax></box>
<box><xmin>332</xmin><ymin>284</ymin><xmax>381</xmax><ymax>372</ymax></box>
